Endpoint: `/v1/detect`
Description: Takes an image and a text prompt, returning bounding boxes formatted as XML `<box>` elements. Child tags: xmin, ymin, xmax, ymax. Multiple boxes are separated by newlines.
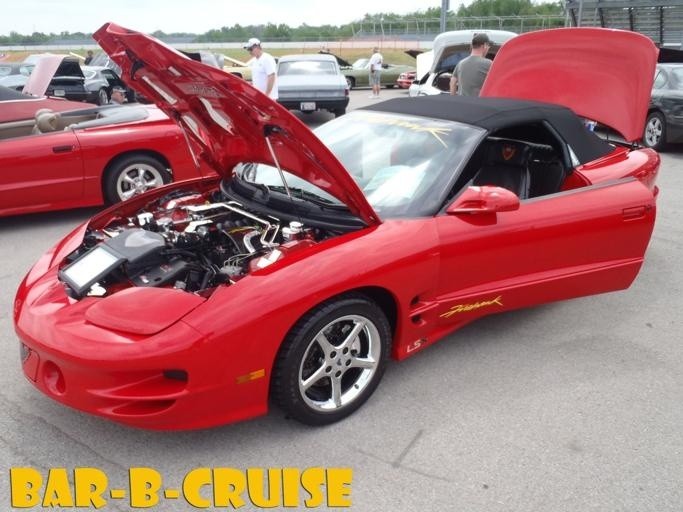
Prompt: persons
<box><xmin>449</xmin><ymin>32</ymin><xmax>494</xmax><ymax>96</ymax></box>
<box><xmin>84</xmin><ymin>50</ymin><xmax>94</xmax><ymax>65</ymax></box>
<box><xmin>465</xmin><ymin>134</ymin><xmax>531</xmax><ymax>201</ymax></box>
<box><xmin>108</xmin><ymin>85</ymin><xmax>127</xmax><ymax>106</ymax></box>
<box><xmin>368</xmin><ymin>45</ymin><xmax>383</xmax><ymax>99</ymax></box>
<box><xmin>243</xmin><ymin>38</ymin><xmax>278</xmax><ymax>103</ymax></box>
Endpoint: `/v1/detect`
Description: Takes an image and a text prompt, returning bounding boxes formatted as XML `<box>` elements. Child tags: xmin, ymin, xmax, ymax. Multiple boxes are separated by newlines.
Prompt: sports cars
<box><xmin>7</xmin><ymin>14</ymin><xmax>664</xmax><ymax>435</ymax></box>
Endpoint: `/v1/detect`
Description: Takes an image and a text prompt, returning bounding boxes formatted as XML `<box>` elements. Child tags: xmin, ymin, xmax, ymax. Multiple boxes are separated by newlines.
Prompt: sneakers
<box><xmin>368</xmin><ymin>94</ymin><xmax>380</xmax><ymax>99</ymax></box>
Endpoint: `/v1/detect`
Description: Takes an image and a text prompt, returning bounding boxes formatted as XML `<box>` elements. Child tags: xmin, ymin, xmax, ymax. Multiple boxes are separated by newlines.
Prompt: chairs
<box><xmin>472</xmin><ymin>138</ymin><xmax>536</xmax><ymax>205</ymax></box>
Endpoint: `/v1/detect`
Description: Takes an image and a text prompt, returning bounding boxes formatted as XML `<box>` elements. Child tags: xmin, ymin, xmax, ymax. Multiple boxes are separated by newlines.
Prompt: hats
<box><xmin>373</xmin><ymin>46</ymin><xmax>379</xmax><ymax>51</ymax></box>
<box><xmin>242</xmin><ymin>38</ymin><xmax>261</xmax><ymax>49</ymax></box>
